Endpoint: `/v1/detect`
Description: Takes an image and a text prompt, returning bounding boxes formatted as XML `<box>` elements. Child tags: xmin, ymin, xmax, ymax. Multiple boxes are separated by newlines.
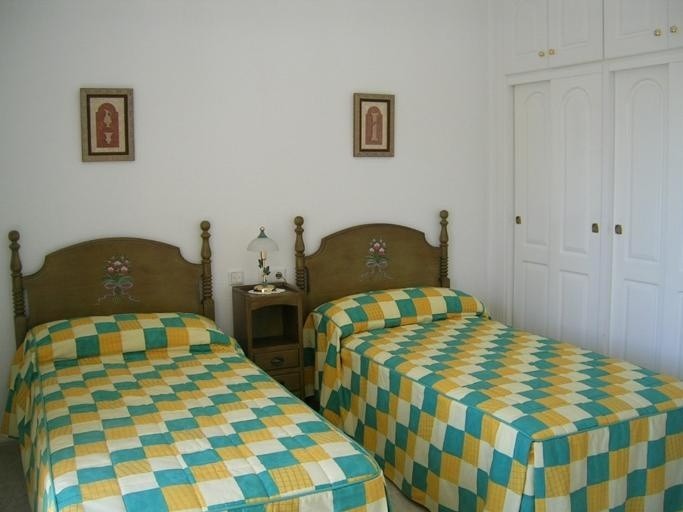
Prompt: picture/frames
<box><xmin>80</xmin><ymin>88</ymin><xmax>135</xmax><ymax>162</ymax></box>
<box><xmin>353</xmin><ymin>93</ymin><xmax>395</xmax><ymax>157</ymax></box>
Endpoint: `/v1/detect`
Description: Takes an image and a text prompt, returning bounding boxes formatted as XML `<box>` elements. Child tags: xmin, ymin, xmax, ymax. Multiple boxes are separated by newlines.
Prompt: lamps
<box><xmin>247</xmin><ymin>225</ymin><xmax>279</xmax><ymax>294</ymax></box>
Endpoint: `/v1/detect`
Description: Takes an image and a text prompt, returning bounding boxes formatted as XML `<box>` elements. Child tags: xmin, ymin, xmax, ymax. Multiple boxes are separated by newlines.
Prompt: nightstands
<box><xmin>232</xmin><ymin>279</ymin><xmax>306</xmax><ymax>402</ymax></box>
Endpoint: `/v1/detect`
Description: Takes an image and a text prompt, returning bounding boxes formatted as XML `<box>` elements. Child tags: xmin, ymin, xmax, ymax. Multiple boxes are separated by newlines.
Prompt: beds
<box><xmin>1</xmin><ymin>221</ymin><xmax>393</xmax><ymax>511</ymax></box>
<box><xmin>293</xmin><ymin>209</ymin><xmax>683</xmax><ymax>512</ymax></box>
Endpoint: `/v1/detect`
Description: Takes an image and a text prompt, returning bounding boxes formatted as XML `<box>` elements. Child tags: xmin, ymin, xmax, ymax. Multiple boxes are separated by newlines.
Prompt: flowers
<box><xmin>366</xmin><ymin>238</ymin><xmax>387</xmax><ymax>274</ymax></box>
<box><xmin>101</xmin><ymin>253</ymin><xmax>131</xmax><ymax>297</ymax></box>
